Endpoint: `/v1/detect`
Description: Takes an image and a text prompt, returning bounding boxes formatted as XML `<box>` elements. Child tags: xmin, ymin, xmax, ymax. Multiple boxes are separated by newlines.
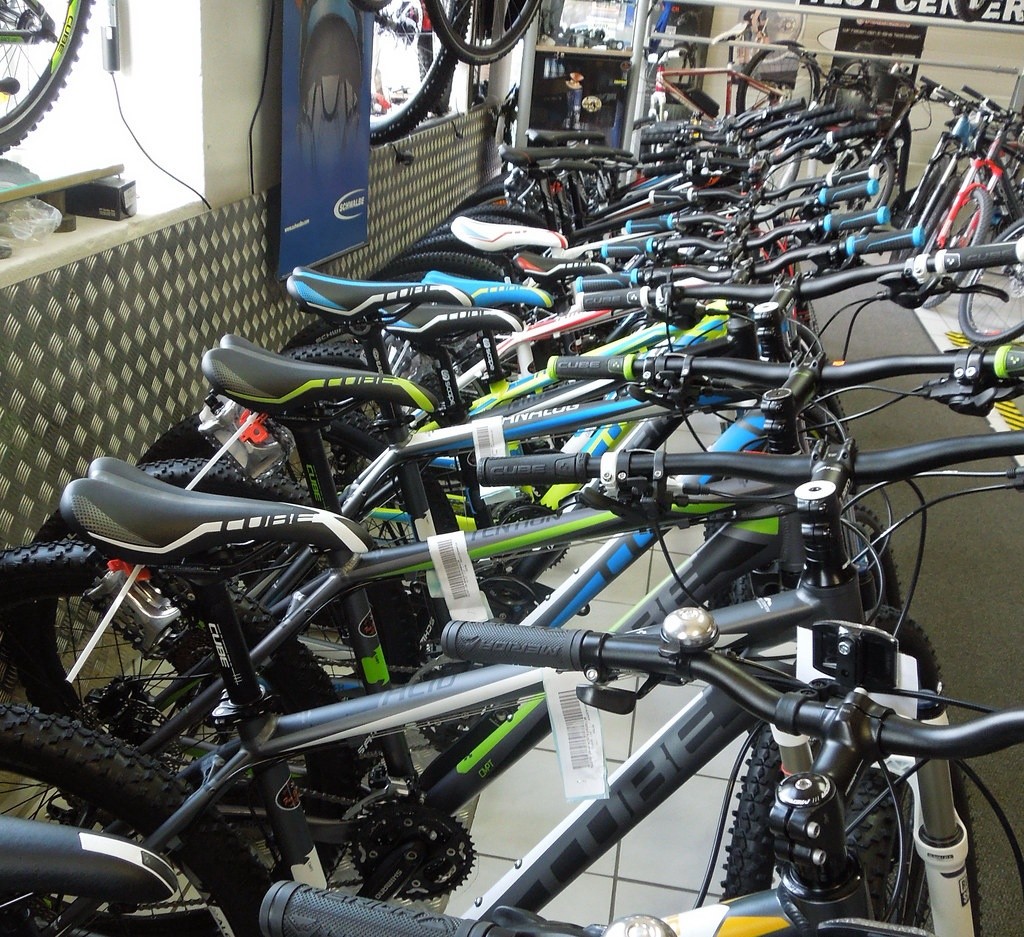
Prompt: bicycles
<box><xmin>1</xmin><ymin>22</ymin><xmax>1024</xmax><ymax>937</ymax></box>
<box><xmin>366</xmin><ymin>1</ymin><xmax>541</xmax><ymax>146</ymax></box>
